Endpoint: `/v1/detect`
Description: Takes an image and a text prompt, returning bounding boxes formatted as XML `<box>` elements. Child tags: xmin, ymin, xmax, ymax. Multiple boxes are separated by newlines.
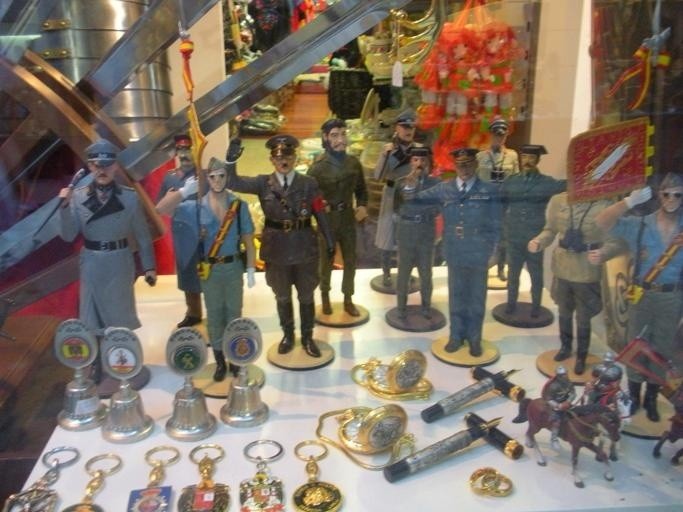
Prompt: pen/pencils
<box><xmin>384</xmin><ymin>416</ymin><xmax>504</xmax><ymax>483</ymax></box>
<box><xmin>422</xmin><ymin>368</ymin><xmax>524</xmax><ymax>422</ymax></box>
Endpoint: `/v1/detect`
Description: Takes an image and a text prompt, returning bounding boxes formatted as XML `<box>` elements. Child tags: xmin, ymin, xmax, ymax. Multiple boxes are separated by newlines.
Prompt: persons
<box><xmin>306</xmin><ymin>119</ymin><xmax>370</xmax><ymax>314</ymax></box>
<box><xmin>57</xmin><ymin>141</ymin><xmax>160</xmax><ymax>384</ymax></box>
<box><xmin>157</xmin><ymin>130</ymin><xmax>213</xmax><ymax>325</ymax></box>
<box><xmin>153</xmin><ymin>159</ymin><xmax>256</xmax><ymax>383</ymax></box>
<box><xmin>223</xmin><ymin>137</ymin><xmax>333</xmax><ymax>360</ymax></box>
<box><xmin>376</xmin><ymin>107</ymin><xmax>682</xmax><ymax>450</ymax></box>
